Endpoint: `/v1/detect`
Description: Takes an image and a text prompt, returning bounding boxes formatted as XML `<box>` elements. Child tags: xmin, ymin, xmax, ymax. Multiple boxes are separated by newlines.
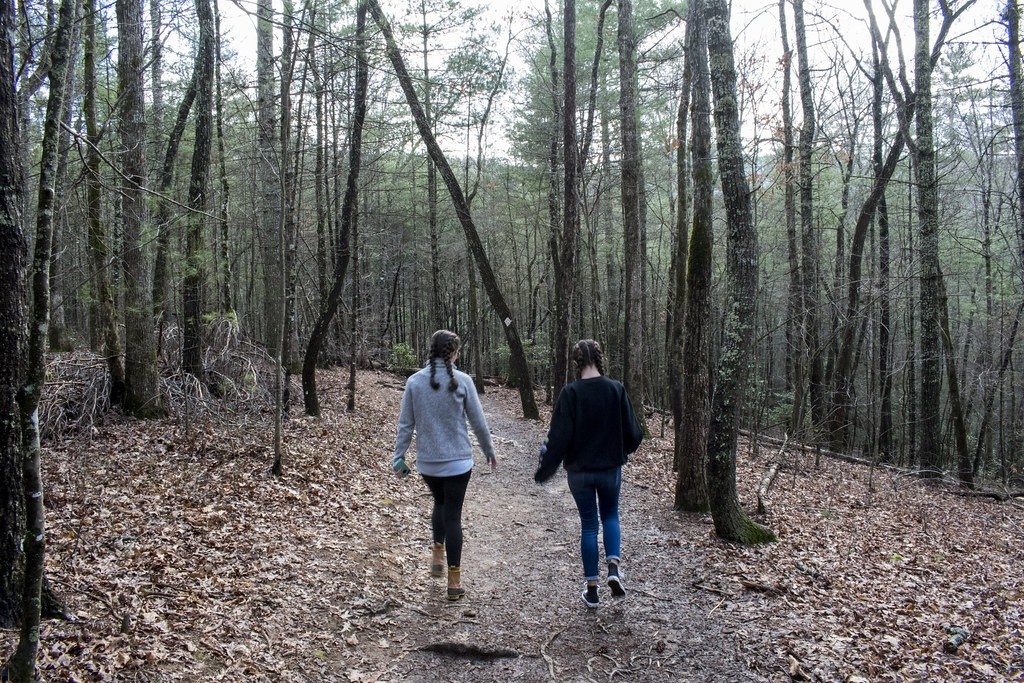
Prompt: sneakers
<box><xmin>582</xmin><ymin>583</ymin><xmax>600</xmax><ymax>608</ymax></box>
<box><xmin>607</xmin><ymin>562</ymin><xmax>626</xmax><ymax>597</ymax></box>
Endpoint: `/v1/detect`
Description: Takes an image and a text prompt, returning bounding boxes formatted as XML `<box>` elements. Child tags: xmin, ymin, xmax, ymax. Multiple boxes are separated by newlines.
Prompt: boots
<box><xmin>432</xmin><ymin>542</ymin><xmax>444</xmax><ymax>576</ymax></box>
<box><xmin>448</xmin><ymin>566</ymin><xmax>466</xmax><ymax>600</ymax></box>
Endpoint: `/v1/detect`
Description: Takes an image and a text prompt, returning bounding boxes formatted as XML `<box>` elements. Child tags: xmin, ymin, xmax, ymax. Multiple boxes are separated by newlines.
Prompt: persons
<box><xmin>394</xmin><ymin>329</ymin><xmax>496</xmax><ymax>600</ymax></box>
<box><xmin>535</xmin><ymin>338</ymin><xmax>644</xmax><ymax>607</ymax></box>
